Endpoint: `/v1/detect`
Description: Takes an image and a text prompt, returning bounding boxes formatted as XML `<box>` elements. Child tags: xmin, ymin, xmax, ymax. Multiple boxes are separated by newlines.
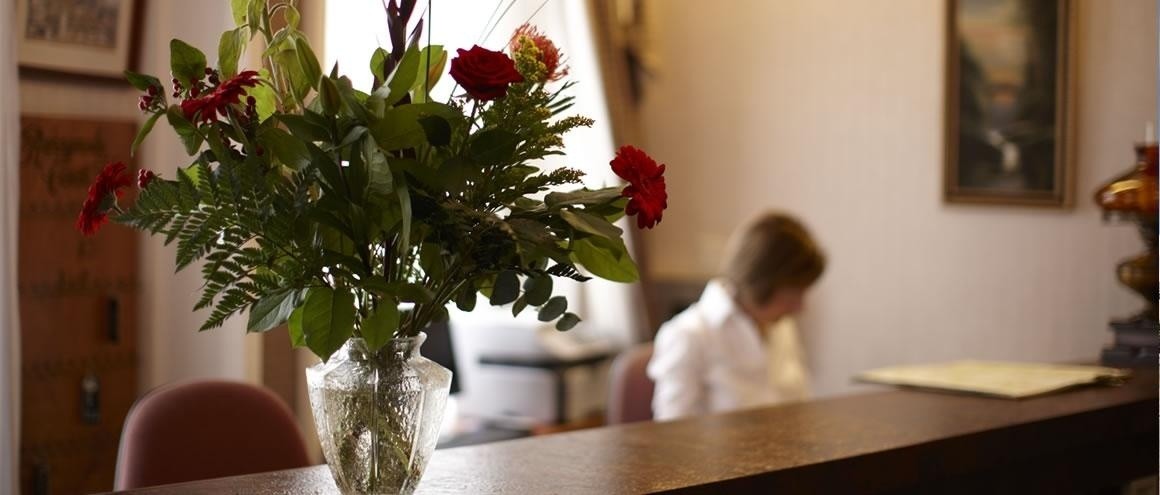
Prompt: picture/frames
<box><xmin>939</xmin><ymin>0</ymin><xmax>1082</xmax><ymax>214</ymax></box>
<box><xmin>15</xmin><ymin>0</ymin><xmax>147</xmax><ymax>88</ymax></box>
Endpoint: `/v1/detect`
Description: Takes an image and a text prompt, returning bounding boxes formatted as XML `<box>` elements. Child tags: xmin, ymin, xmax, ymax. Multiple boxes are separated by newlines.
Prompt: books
<box><xmin>854</xmin><ymin>352</ymin><xmax>1132</xmax><ymax>402</ymax></box>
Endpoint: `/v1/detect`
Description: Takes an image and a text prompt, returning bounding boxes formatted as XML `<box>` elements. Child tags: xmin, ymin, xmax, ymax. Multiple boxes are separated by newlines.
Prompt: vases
<box><xmin>302</xmin><ymin>332</ymin><xmax>456</xmax><ymax>495</ymax></box>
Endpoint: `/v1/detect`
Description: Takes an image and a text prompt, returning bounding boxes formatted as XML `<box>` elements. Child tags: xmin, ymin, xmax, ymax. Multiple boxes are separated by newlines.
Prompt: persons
<box><xmin>639</xmin><ymin>207</ymin><xmax>828</xmax><ymax>428</ymax></box>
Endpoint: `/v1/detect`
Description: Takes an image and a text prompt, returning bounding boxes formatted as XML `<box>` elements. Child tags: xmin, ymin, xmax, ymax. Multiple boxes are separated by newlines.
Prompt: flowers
<box><xmin>77</xmin><ymin>0</ymin><xmax>666</xmax><ymax>494</ymax></box>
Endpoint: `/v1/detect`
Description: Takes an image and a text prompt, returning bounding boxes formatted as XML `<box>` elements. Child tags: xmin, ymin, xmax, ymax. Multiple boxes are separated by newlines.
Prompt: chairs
<box><xmin>605</xmin><ymin>346</ymin><xmax>653</xmax><ymax>425</ymax></box>
<box><xmin>109</xmin><ymin>381</ymin><xmax>312</xmax><ymax>494</ymax></box>
